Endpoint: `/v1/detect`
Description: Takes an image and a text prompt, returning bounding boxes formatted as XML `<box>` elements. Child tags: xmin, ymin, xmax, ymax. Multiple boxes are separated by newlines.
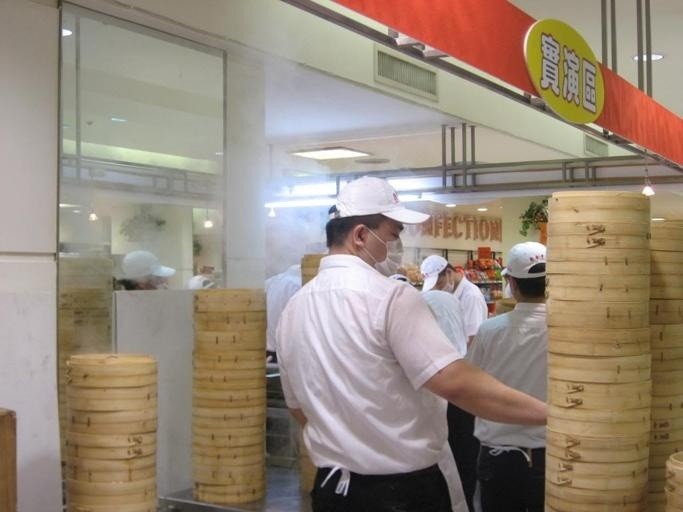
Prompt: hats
<box><xmin>327</xmin><ymin>176</ymin><xmax>431</xmax><ymax>225</ymax></box>
<box><xmin>120</xmin><ymin>249</ymin><xmax>176</xmax><ymax>279</ymax></box>
<box><xmin>501</xmin><ymin>241</ymin><xmax>547</xmax><ymax>279</ymax></box>
<box><xmin>187</xmin><ymin>274</ymin><xmax>215</xmax><ymax>289</ymax></box>
<box><xmin>420</xmin><ymin>254</ymin><xmax>449</xmax><ymax>293</ymax></box>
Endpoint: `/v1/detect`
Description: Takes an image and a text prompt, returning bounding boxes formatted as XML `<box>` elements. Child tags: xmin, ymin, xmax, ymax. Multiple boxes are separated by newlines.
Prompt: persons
<box><xmin>185</xmin><ymin>273</ymin><xmax>220</xmax><ymax>289</ymax></box>
<box><xmin>276</xmin><ymin>178</ymin><xmax>549</xmax><ymax>511</ymax></box>
<box><xmin>262</xmin><ymin>260</ymin><xmax>301</xmax><ymax>458</ymax></box>
<box><xmin>463</xmin><ymin>240</ymin><xmax>548</xmax><ymax>512</ymax></box>
<box><xmin>386</xmin><ymin>273</ymin><xmax>473</xmax><ymax>512</ymax></box>
<box><xmin>417</xmin><ymin>251</ymin><xmax>488</xmax><ymax>353</ymax></box>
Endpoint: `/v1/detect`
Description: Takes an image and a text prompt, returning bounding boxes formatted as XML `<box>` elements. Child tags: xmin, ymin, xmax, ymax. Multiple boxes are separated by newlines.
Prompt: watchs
<box><xmin>116</xmin><ymin>251</ymin><xmax>176</xmax><ymax>291</ymax></box>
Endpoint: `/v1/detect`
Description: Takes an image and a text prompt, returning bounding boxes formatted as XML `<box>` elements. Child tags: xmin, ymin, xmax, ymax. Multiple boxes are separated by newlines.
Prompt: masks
<box><xmin>149</xmin><ymin>283</ymin><xmax>169</xmax><ymax>290</ymax></box>
<box><xmin>361</xmin><ymin>226</ymin><xmax>404</xmax><ymax>277</ymax></box>
<box><xmin>442</xmin><ymin>271</ymin><xmax>455</xmax><ymax>293</ymax></box>
